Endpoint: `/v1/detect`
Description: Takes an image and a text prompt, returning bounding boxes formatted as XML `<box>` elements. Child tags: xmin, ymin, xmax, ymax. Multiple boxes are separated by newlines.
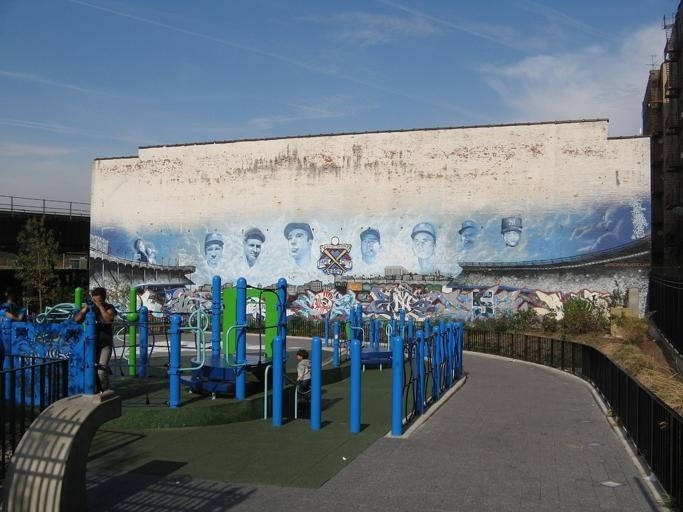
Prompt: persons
<box><xmin>238</xmin><ymin>227</ymin><xmax>266</xmax><ymax>272</ymax></box>
<box><xmin>356</xmin><ymin>226</ymin><xmax>385</xmax><ymax>272</ymax></box>
<box><xmin>457</xmin><ymin>220</ymin><xmax>487</xmax><ymax>254</ymax></box>
<box><xmin>75</xmin><ymin>287</ymin><xmax>117</xmax><ymax>392</ymax></box>
<box><xmin>284</xmin><ymin>222</ymin><xmax>315</xmax><ymax>272</ymax></box>
<box><xmin>0</xmin><ymin>295</ymin><xmax>27</xmax><ymax>321</ymax></box>
<box><xmin>407</xmin><ymin>222</ymin><xmax>447</xmax><ymax>273</ymax></box>
<box><xmin>295</xmin><ymin>349</ymin><xmax>312</xmax><ymax>392</ymax></box>
<box><xmin>500</xmin><ymin>218</ymin><xmax>523</xmax><ymax>258</ymax></box>
<box><xmin>199</xmin><ymin>232</ymin><xmax>227</xmax><ymax>272</ymax></box>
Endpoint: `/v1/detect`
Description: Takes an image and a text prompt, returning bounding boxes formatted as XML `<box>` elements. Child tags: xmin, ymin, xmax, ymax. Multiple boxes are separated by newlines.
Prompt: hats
<box><xmin>283</xmin><ymin>222</ymin><xmax>315</xmax><ymax>240</ymax></box>
<box><xmin>410</xmin><ymin>223</ymin><xmax>437</xmax><ymax>240</ymax></box>
<box><xmin>458</xmin><ymin>220</ymin><xmax>476</xmax><ymax>234</ymax></box>
<box><xmin>501</xmin><ymin>216</ymin><xmax>525</xmax><ymax>235</ymax></box>
<box><xmin>360</xmin><ymin>225</ymin><xmax>381</xmax><ymax>238</ymax></box>
<box><xmin>244</xmin><ymin>227</ymin><xmax>266</xmax><ymax>243</ymax></box>
<box><xmin>204</xmin><ymin>232</ymin><xmax>225</xmax><ymax>246</ymax></box>
